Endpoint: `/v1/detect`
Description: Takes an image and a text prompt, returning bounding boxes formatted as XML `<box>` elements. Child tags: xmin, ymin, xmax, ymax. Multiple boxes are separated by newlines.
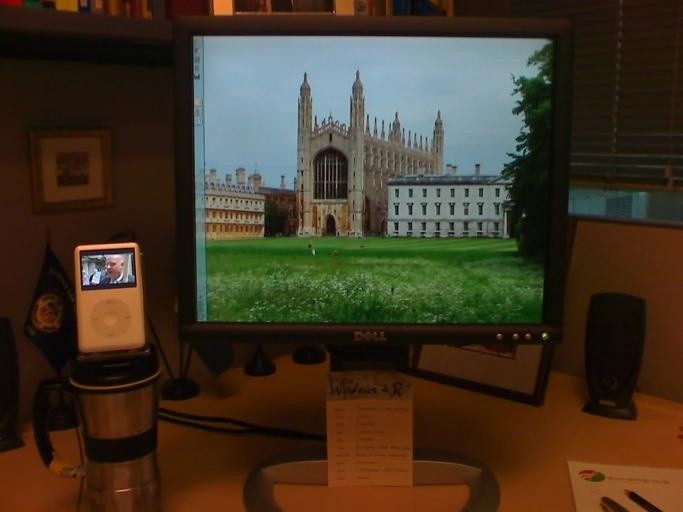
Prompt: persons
<box><xmin>98</xmin><ymin>255</ymin><xmax>135</xmax><ymax>284</ymax></box>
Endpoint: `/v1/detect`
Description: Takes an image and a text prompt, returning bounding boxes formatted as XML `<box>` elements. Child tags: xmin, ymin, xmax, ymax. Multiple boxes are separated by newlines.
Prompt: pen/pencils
<box><xmin>601</xmin><ymin>488</ymin><xmax>662</xmax><ymax>512</ymax></box>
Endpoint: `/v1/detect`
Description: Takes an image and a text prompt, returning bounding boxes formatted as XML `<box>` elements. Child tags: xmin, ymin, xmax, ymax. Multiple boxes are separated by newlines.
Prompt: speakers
<box><xmin>0</xmin><ymin>317</ymin><xmax>25</xmax><ymax>452</ymax></box>
<box><xmin>581</xmin><ymin>292</ymin><xmax>646</xmax><ymax>420</ymax></box>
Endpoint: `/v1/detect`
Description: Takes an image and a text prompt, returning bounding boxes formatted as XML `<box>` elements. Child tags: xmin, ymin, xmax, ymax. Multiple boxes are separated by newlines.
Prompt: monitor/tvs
<box><xmin>172</xmin><ymin>14</ymin><xmax>574</xmax><ymax>512</ymax></box>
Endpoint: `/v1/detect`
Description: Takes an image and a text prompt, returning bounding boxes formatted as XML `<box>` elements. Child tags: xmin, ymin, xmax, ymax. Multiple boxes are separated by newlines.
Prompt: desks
<box><xmin>0</xmin><ymin>350</ymin><xmax>683</xmax><ymax>512</ymax></box>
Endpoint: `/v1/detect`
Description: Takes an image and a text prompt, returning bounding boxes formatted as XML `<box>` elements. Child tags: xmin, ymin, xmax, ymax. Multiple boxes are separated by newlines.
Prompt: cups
<box><xmin>32</xmin><ymin>366</ymin><xmax>162</xmax><ymax>512</ymax></box>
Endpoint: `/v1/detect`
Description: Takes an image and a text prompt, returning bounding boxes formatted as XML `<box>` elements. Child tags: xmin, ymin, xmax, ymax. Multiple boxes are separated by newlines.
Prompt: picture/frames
<box><xmin>409</xmin><ymin>215</ymin><xmax>578</xmax><ymax>407</ymax></box>
<box><xmin>29</xmin><ymin>127</ymin><xmax>113</xmax><ymax>214</ymax></box>
